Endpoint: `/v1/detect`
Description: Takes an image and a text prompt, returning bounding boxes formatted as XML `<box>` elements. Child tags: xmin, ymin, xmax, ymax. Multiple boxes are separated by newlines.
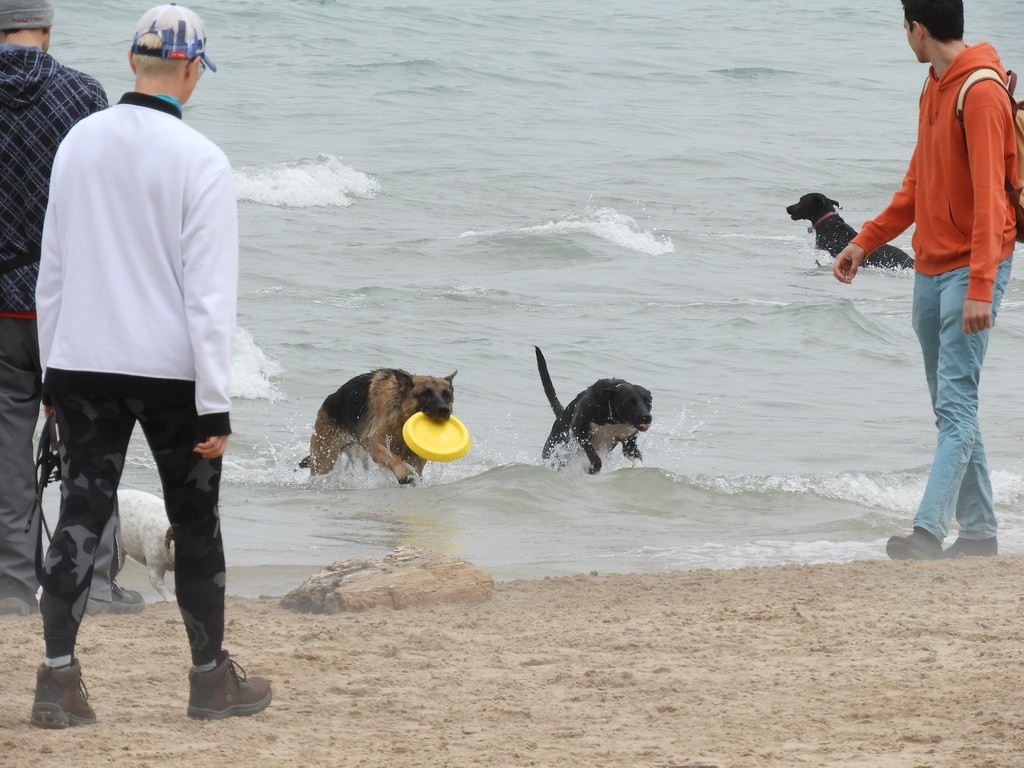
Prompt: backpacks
<box><xmin>956</xmin><ymin>69</ymin><xmax>1024</xmax><ymax>244</ymax></box>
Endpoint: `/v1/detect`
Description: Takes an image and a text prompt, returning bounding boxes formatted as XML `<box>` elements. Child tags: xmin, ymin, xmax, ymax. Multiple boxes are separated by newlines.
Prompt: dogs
<box><xmin>117</xmin><ymin>489</ymin><xmax>177</xmax><ymax>605</ymax></box>
<box><xmin>786</xmin><ymin>192</ymin><xmax>914</xmax><ymax>271</ymax></box>
<box><xmin>294</xmin><ymin>369</ymin><xmax>459</xmax><ymax>487</ymax></box>
<box><xmin>535</xmin><ymin>345</ymin><xmax>653</xmax><ymax>475</ymax></box>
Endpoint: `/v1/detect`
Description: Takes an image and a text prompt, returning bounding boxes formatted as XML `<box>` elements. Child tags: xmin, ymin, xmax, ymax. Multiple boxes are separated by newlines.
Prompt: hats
<box><xmin>0</xmin><ymin>1</ymin><xmax>57</xmax><ymax>30</ymax></box>
<box><xmin>130</xmin><ymin>2</ymin><xmax>217</xmax><ymax>73</ymax></box>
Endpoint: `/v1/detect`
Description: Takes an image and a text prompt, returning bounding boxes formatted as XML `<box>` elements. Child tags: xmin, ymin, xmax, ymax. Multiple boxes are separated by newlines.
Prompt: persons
<box><xmin>0</xmin><ymin>0</ymin><xmax>146</xmax><ymax>616</ymax></box>
<box><xmin>31</xmin><ymin>3</ymin><xmax>273</xmax><ymax>729</ymax></box>
<box><xmin>834</xmin><ymin>0</ymin><xmax>1024</xmax><ymax>561</ymax></box>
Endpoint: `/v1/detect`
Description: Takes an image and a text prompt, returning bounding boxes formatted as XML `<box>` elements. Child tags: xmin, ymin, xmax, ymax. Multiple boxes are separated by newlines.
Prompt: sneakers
<box><xmin>186</xmin><ymin>649</ymin><xmax>273</xmax><ymax>718</ymax></box>
<box><xmin>885</xmin><ymin>527</ymin><xmax>943</xmax><ymax>560</ymax></box>
<box><xmin>85</xmin><ymin>582</ymin><xmax>148</xmax><ymax>615</ymax></box>
<box><xmin>0</xmin><ymin>576</ymin><xmax>39</xmax><ymax>615</ymax></box>
<box><xmin>943</xmin><ymin>539</ymin><xmax>998</xmax><ymax>556</ymax></box>
<box><xmin>29</xmin><ymin>660</ymin><xmax>97</xmax><ymax>729</ymax></box>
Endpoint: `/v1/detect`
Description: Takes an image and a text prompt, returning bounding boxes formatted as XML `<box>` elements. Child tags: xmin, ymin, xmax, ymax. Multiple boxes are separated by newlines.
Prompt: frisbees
<box><xmin>401</xmin><ymin>410</ymin><xmax>473</xmax><ymax>463</ymax></box>
